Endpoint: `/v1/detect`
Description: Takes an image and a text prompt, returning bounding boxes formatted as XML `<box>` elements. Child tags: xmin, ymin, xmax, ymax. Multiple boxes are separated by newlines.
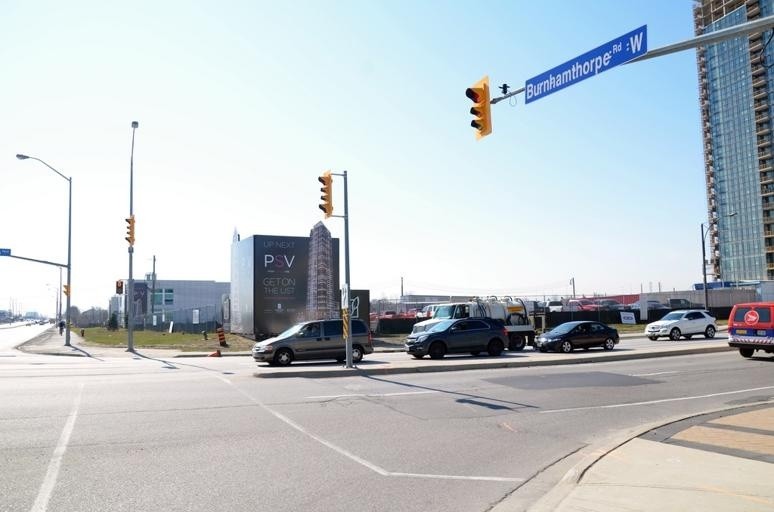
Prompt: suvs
<box><xmin>644</xmin><ymin>308</ymin><xmax>716</xmax><ymax>339</ymax></box>
<box><xmin>728</xmin><ymin>302</ymin><xmax>773</xmax><ymax>357</ymax></box>
<box><xmin>405</xmin><ymin>316</ymin><xmax>509</xmax><ymax>359</ymax></box>
<box><xmin>252</xmin><ymin>315</ymin><xmax>373</xmax><ymax>366</ymax></box>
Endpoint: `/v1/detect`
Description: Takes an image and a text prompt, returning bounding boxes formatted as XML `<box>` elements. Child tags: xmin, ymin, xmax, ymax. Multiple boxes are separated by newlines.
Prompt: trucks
<box><xmin>409</xmin><ymin>299</ymin><xmax>535</xmax><ymax>351</ymax></box>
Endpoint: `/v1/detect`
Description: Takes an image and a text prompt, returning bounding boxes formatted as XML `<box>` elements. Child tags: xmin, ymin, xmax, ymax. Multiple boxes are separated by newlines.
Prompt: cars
<box><xmin>534</xmin><ymin>320</ymin><xmax>618</xmax><ymax>353</ymax></box>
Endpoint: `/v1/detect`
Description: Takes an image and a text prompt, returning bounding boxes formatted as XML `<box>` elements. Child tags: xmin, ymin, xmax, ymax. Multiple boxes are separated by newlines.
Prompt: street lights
<box><xmin>127</xmin><ymin>120</ymin><xmax>139</xmax><ymax>354</ymax></box>
<box><xmin>700</xmin><ymin>212</ymin><xmax>738</xmax><ymax>315</ymax></box>
<box><xmin>14</xmin><ymin>154</ymin><xmax>73</xmax><ymax>346</ymax></box>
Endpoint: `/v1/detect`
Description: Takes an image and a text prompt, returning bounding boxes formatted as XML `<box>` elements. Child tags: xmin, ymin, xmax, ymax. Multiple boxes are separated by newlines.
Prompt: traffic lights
<box><xmin>317</xmin><ymin>177</ymin><xmax>332</xmax><ymax>215</ymax></box>
<box><xmin>465</xmin><ymin>74</ymin><xmax>492</xmax><ymax>137</ymax></box>
<box><xmin>124</xmin><ymin>217</ymin><xmax>135</xmax><ymax>242</ymax></box>
<box><xmin>115</xmin><ymin>280</ymin><xmax>124</xmax><ymax>295</ymax></box>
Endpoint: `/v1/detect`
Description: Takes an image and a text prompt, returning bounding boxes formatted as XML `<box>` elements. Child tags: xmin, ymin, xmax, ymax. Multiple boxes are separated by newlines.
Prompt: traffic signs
<box><xmin>0</xmin><ymin>248</ymin><xmax>11</xmax><ymax>257</ymax></box>
<box><xmin>525</xmin><ymin>24</ymin><xmax>650</xmax><ymax>102</ymax></box>
